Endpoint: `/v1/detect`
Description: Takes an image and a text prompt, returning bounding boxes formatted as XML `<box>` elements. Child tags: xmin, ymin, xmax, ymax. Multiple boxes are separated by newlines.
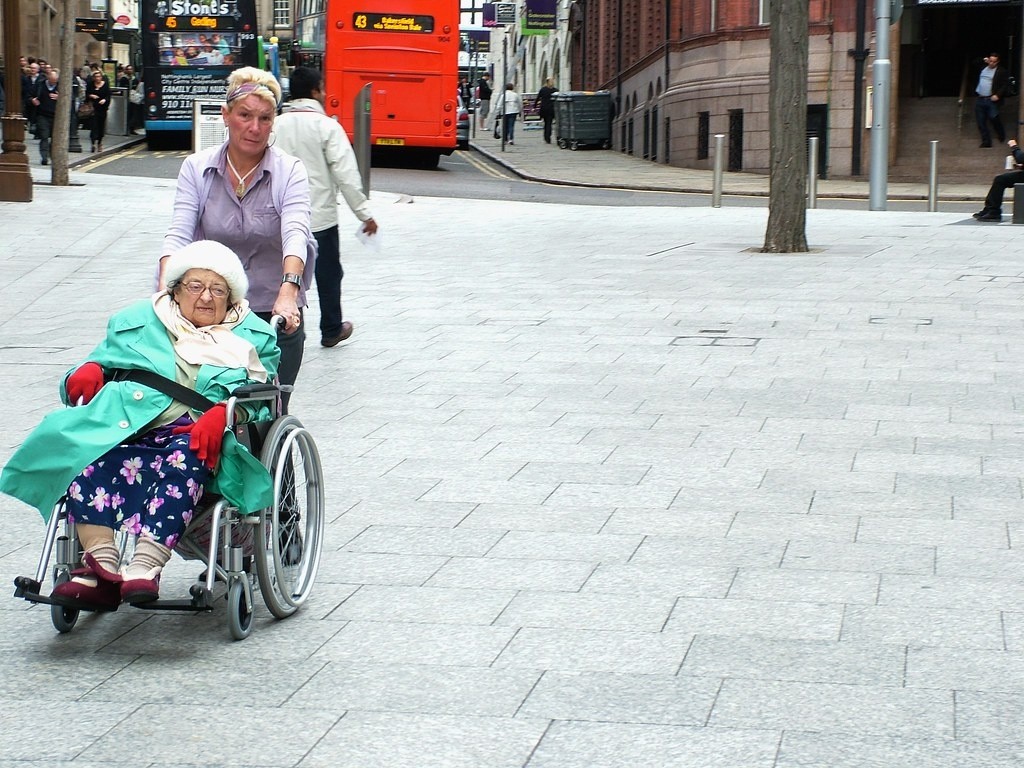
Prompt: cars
<box><xmin>456</xmin><ymin>95</ymin><xmax>470</xmax><ymax>150</ymax></box>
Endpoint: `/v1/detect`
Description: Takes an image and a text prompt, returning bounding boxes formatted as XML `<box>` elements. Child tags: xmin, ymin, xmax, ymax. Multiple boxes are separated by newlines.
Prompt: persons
<box><xmin>971</xmin><ymin>51</ymin><xmax>1007</xmax><ymax>148</ymax></box>
<box><xmin>972</xmin><ymin>118</ymin><xmax>1024</xmax><ymax>223</ymax></box>
<box><xmin>531</xmin><ymin>77</ymin><xmax>560</xmax><ymax>145</ymax></box>
<box><xmin>172</xmin><ymin>34</ymin><xmax>238</xmax><ymax>64</ymax></box>
<box><xmin>1</xmin><ymin>243</ymin><xmax>283</xmax><ymax>613</ymax></box>
<box><xmin>156</xmin><ymin>64</ymin><xmax>319</xmax><ymax>583</ymax></box>
<box><xmin>269</xmin><ymin>65</ymin><xmax>377</xmax><ymax>347</ymax></box>
<box><xmin>479</xmin><ymin>72</ymin><xmax>491</xmax><ymax>131</ymax></box>
<box><xmin>459</xmin><ymin>77</ymin><xmax>473</xmax><ymax>112</ymax></box>
<box><xmin>19</xmin><ymin>52</ymin><xmax>61</xmax><ymax>167</ymax></box>
<box><xmin>116</xmin><ymin>61</ymin><xmax>145</xmax><ymax>136</ymax></box>
<box><xmin>70</xmin><ymin>57</ymin><xmax>111</xmax><ymax>153</ymax></box>
<box><xmin>496</xmin><ymin>83</ymin><xmax>522</xmax><ymax>144</ymax></box>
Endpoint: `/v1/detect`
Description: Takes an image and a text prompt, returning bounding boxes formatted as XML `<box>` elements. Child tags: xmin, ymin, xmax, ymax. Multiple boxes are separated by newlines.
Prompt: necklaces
<box><xmin>227</xmin><ymin>149</ymin><xmax>265</xmax><ymax>199</ymax></box>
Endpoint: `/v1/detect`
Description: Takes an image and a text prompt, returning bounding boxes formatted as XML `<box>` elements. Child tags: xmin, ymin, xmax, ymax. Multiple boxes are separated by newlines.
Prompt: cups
<box><xmin>1005</xmin><ymin>155</ymin><xmax>1015</xmax><ymax>169</ymax></box>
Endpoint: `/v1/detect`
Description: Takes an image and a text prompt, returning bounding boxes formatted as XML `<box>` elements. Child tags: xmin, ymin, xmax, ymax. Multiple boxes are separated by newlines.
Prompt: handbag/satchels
<box><xmin>78</xmin><ymin>102</ymin><xmax>95</xmax><ymax>118</ymax></box>
<box><xmin>494</xmin><ymin>120</ymin><xmax>502</xmax><ymax>138</ymax></box>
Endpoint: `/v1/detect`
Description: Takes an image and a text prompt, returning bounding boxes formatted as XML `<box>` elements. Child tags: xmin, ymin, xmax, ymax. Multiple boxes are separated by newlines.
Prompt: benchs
<box><xmin>1013</xmin><ymin>183</ymin><xmax>1024</xmax><ymax>224</ymax></box>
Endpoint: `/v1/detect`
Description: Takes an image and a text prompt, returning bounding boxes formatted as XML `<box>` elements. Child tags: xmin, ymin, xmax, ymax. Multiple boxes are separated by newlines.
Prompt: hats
<box><xmin>165</xmin><ymin>239</ymin><xmax>249</xmax><ymax>304</ymax></box>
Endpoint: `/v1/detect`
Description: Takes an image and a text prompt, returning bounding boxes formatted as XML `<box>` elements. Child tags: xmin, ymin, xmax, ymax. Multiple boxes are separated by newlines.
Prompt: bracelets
<box><xmin>368</xmin><ymin>218</ymin><xmax>375</xmax><ymax>222</ymax></box>
<box><xmin>280</xmin><ymin>272</ymin><xmax>302</xmax><ymax>290</ymax></box>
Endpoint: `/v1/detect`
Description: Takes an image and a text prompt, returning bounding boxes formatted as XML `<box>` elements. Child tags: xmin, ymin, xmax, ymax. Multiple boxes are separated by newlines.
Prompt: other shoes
<box><xmin>51</xmin><ymin>567</ymin><xmax>119</xmax><ymax>608</ymax></box>
<box><xmin>42</xmin><ymin>157</ymin><xmax>50</xmax><ymax>165</ymax></box>
<box><xmin>98</xmin><ymin>144</ymin><xmax>103</xmax><ymax>152</ymax></box>
<box><xmin>321</xmin><ymin>322</ymin><xmax>352</xmax><ymax>346</ymax></box>
<box><xmin>91</xmin><ymin>146</ymin><xmax>95</xmax><ymax>153</ymax></box>
<box><xmin>509</xmin><ymin>141</ymin><xmax>513</xmax><ymax>145</ymax></box>
<box><xmin>120</xmin><ymin>579</ymin><xmax>158</xmax><ymax>601</ymax></box>
<box><xmin>480</xmin><ymin>127</ymin><xmax>489</xmax><ymax>130</ymax></box>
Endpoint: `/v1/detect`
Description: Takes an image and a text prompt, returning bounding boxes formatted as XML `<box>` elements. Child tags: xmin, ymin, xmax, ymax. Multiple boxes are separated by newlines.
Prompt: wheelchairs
<box><xmin>12</xmin><ymin>313</ymin><xmax>326</xmax><ymax>641</ymax></box>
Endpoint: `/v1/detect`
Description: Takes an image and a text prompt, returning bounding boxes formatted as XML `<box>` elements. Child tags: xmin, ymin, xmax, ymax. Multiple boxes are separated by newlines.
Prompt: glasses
<box><xmin>181</xmin><ymin>281</ymin><xmax>231</xmax><ymax>298</ymax></box>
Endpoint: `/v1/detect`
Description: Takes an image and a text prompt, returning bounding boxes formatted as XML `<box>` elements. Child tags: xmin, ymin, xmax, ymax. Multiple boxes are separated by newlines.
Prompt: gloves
<box><xmin>67</xmin><ymin>362</ymin><xmax>104</xmax><ymax>406</ymax></box>
<box><xmin>172</xmin><ymin>404</ymin><xmax>236</xmax><ymax>468</ymax></box>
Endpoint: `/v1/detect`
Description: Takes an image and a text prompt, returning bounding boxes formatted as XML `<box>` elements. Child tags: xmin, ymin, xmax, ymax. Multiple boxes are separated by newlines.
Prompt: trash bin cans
<box><xmin>103</xmin><ymin>87</ymin><xmax>129</xmax><ymax>137</ymax></box>
<box><xmin>552</xmin><ymin>88</ymin><xmax>613</xmax><ymax>150</ymax></box>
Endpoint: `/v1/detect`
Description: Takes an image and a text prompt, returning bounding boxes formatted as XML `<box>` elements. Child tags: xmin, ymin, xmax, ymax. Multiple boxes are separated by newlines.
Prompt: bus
<box><xmin>257</xmin><ymin>34</ymin><xmax>282</xmax><ymax>82</ymax></box>
<box><xmin>139</xmin><ymin>0</ymin><xmax>258</xmax><ymax>153</ymax></box>
<box><xmin>287</xmin><ymin>0</ymin><xmax>458</xmax><ymax>170</ymax></box>
